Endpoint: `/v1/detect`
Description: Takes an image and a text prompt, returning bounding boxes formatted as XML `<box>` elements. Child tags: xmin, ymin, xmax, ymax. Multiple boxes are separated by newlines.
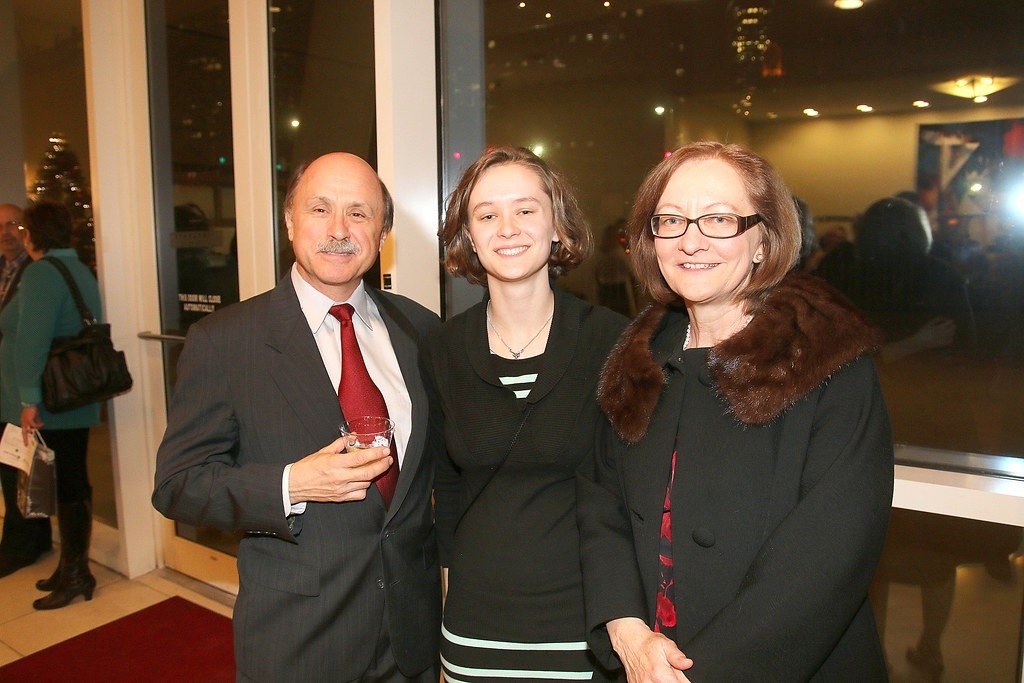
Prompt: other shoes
<box><xmin>0</xmin><ymin>538</ymin><xmax>53</xmax><ymax>578</ymax></box>
<box><xmin>905</xmin><ymin>639</ymin><xmax>945</xmax><ymax>674</ymax></box>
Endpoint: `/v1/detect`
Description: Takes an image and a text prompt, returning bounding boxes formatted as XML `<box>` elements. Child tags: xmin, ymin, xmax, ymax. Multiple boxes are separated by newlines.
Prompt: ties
<box><xmin>329</xmin><ymin>304</ymin><xmax>400</xmax><ymax>512</ymax></box>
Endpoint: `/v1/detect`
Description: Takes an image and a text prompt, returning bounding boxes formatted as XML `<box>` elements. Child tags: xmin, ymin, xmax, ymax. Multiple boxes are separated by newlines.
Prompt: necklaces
<box><xmin>487</xmin><ymin>299</ymin><xmax>552</xmax><ymax>359</ymax></box>
<box><xmin>683</xmin><ymin>324</ymin><xmax>690</xmax><ymax>349</ymax></box>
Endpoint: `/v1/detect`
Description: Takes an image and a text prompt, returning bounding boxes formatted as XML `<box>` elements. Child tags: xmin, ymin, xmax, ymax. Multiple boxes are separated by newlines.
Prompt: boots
<box><xmin>33</xmin><ymin>497</ymin><xmax>96</xmax><ymax>609</ymax></box>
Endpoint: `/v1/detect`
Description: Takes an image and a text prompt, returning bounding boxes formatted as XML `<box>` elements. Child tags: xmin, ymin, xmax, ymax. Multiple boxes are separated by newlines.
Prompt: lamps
<box><xmin>927</xmin><ymin>61</ymin><xmax>1024</xmax><ymax>99</ymax></box>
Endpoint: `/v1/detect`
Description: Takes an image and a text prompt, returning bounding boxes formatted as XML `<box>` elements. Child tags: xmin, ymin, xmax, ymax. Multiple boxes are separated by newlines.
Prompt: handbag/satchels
<box><xmin>36</xmin><ymin>255</ymin><xmax>133</xmax><ymax>412</ymax></box>
<box><xmin>17</xmin><ymin>429</ymin><xmax>57</xmax><ymax>518</ymax></box>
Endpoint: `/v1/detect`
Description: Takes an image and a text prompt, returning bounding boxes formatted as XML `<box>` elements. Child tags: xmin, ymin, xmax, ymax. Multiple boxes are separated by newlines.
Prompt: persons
<box><xmin>0</xmin><ymin>205</ymin><xmax>55</xmax><ymax>575</ymax></box>
<box><xmin>433</xmin><ymin>145</ymin><xmax>638</xmax><ymax>683</ymax></box>
<box><xmin>0</xmin><ymin>203</ymin><xmax>109</xmax><ymax>611</ymax></box>
<box><xmin>853</xmin><ymin>195</ymin><xmax>981</xmax><ymax>676</ymax></box>
<box><xmin>576</xmin><ymin>142</ymin><xmax>894</xmax><ymax>683</ymax></box>
<box><xmin>594</xmin><ymin>188</ymin><xmax>1024</xmax><ymax>457</ymax></box>
<box><xmin>153</xmin><ymin>152</ymin><xmax>445</xmax><ymax>683</ymax></box>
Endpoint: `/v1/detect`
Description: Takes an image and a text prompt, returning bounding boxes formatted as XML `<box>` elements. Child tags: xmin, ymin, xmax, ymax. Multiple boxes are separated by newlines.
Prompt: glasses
<box><xmin>0</xmin><ymin>221</ymin><xmax>20</xmax><ymax>231</ymax></box>
<box><xmin>650</xmin><ymin>214</ymin><xmax>763</xmax><ymax>239</ymax></box>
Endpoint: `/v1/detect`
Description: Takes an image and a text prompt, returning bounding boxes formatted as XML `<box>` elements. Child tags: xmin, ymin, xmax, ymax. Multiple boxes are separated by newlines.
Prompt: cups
<box><xmin>339</xmin><ymin>416</ymin><xmax>395</xmax><ymax>468</ymax></box>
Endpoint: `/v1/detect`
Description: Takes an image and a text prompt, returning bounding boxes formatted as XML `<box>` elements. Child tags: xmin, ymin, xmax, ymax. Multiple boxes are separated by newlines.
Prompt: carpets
<box><xmin>0</xmin><ymin>595</ymin><xmax>236</xmax><ymax>683</ymax></box>
